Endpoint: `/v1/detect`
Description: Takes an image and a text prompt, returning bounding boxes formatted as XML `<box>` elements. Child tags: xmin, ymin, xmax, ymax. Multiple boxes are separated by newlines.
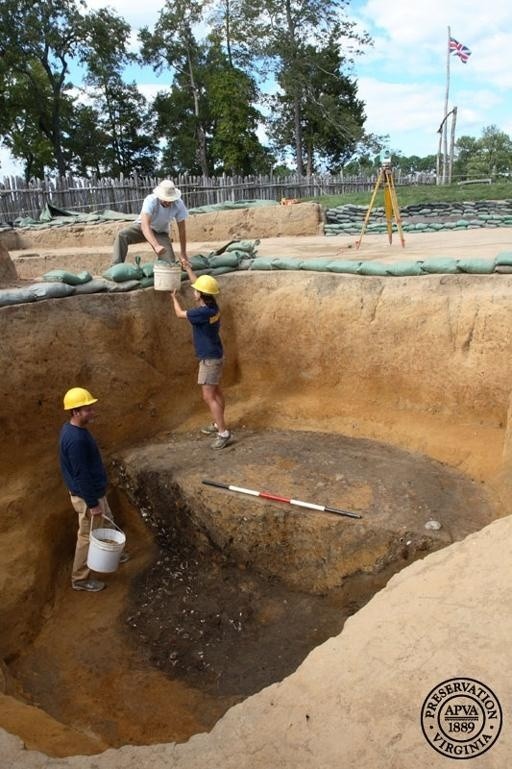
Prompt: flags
<box><xmin>449</xmin><ymin>38</ymin><xmax>472</xmax><ymax>64</ymax></box>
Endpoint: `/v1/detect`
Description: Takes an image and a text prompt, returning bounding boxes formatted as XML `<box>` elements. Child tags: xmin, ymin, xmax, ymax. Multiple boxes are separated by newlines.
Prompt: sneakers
<box><xmin>200</xmin><ymin>423</ymin><xmax>219</xmax><ymax>435</ymax></box>
<box><xmin>71</xmin><ymin>577</ymin><xmax>106</xmax><ymax>593</ymax></box>
<box><xmin>210</xmin><ymin>430</ymin><xmax>234</xmax><ymax>450</ymax></box>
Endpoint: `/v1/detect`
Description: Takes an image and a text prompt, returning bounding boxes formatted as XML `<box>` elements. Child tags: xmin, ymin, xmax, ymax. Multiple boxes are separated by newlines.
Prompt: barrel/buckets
<box><xmin>154</xmin><ymin>258</ymin><xmax>182</xmax><ymax>291</ymax></box>
<box><xmin>87</xmin><ymin>513</ymin><xmax>127</xmax><ymax>573</ymax></box>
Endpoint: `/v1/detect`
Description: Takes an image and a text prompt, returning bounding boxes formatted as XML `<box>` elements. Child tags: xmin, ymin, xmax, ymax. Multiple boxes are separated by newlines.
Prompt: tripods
<box><xmin>356</xmin><ymin>166</ymin><xmax>406</xmax><ymax>249</ymax></box>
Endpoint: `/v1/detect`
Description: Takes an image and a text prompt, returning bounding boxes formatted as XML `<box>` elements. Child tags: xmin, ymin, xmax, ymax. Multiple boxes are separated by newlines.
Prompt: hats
<box><xmin>189</xmin><ymin>274</ymin><xmax>220</xmax><ymax>296</ymax></box>
<box><xmin>152</xmin><ymin>178</ymin><xmax>182</xmax><ymax>202</ymax></box>
<box><xmin>63</xmin><ymin>386</ymin><xmax>98</xmax><ymax>411</ymax></box>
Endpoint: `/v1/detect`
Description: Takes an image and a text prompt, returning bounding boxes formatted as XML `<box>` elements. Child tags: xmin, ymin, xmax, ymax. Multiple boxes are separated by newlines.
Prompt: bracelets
<box><xmin>180</xmin><ymin>258</ymin><xmax>186</xmax><ymax>262</ymax></box>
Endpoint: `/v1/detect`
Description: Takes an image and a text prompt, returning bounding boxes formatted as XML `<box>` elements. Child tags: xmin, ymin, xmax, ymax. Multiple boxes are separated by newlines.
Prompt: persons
<box><xmin>169</xmin><ymin>259</ymin><xmax>233</xmax><ymax>450</ymax></box>
<box><xmin>110</xmin><ymin>180</ymin><xmax>189</xmax><ymax>267</ymax></box>
<box><xmin>59</xmin><ymin>387</ymin><xmax>130</xmax><ymax>591</ymax></box>
